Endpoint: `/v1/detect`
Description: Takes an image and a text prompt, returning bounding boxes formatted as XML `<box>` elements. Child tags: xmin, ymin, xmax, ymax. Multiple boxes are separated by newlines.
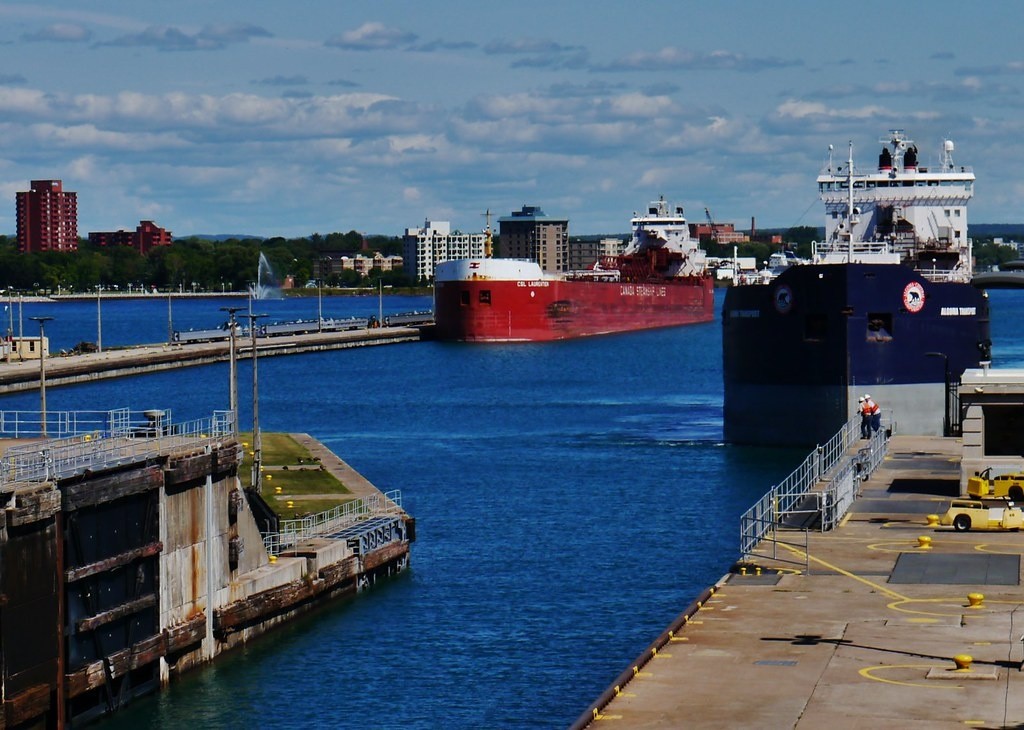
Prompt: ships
<box><xmin>433</xmin><ymin>196</ymin><xmax>715</xmax><ymax>342</ymax></box>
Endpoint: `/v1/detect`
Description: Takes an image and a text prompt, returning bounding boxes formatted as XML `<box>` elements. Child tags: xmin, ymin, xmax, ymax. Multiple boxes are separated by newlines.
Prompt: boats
<box><xmin>723</xmin><ymin>128</ymin><xmax>994</xmax><ymax>448</ymax></box>
<box><xmin>1</xmin><ymin>306</ymin><xmax>418</xmax><ymax>729</ymax></box>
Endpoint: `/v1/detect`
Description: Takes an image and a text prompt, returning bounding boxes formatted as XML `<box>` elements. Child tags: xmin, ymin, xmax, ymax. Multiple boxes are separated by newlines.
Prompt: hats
<box><xmin>860</xmin><ymin>397</ymin><xmax>865</xmax><ymax>401</ymax></box>
<box><xmin>865</xmin><ymin>394</ymin><xmax>870</xmax><ymax>399</ymax></box>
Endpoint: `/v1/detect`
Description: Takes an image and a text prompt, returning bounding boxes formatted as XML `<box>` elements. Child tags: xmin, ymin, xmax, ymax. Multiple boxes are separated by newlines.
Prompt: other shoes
<box><xmin>860</xmin><ymin>436</ymin><xmax>871</xmax><ymax>439</ymax></box>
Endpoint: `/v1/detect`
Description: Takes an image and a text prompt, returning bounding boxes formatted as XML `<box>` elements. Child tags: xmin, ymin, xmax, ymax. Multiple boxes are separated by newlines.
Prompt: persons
<box><xmin>858</xmin><ymin>394</ymin><xmax>881</xmax><ymax>439</ymax></box>
